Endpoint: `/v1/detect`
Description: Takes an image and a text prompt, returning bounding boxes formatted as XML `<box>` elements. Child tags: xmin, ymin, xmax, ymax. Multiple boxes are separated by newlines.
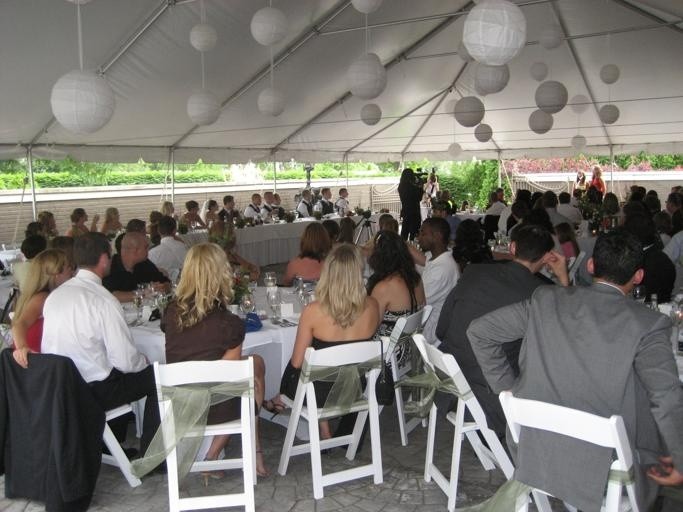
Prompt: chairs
<box><xmin>277</xmin><ymin>337</ymin><xmax>391</xmax><ymax>500</ymax></box>
<box><xmin>152</xmin><ymin>356</ymin><xmax>258</xmax><ymax>512</ymax></box>
<box><xmin>411</xmin><ymin>333</ymin><xmax>514</xmax><ymax>512</ymax></box>
<box><xmin>1</xmin><ymin>347</ymin><xmax>142</xmax><ymax>488</ymax></box>
<box><xmin>499</xmin><ymin>390</ymin><xmax>641</xmax><ymax>512</ymax></box>
<box><xmin>1</xmin><ymin>207</ymin><xmax>683</xmax><ymax>461</ymax></box>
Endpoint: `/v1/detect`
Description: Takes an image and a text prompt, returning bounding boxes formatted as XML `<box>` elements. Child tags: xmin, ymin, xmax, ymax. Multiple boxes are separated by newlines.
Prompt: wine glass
<box><xmin>238</xmin><ymin>270</ymin><xmax>284</xmax><ymax>329</ymax></box>
<box><xmin>127</xmin><ymin>278</ymin><xmax>177</xmax><ymax>327</ymax></box>
<box><xmin>186</xmin><ymin>208</ymin><xmax>300</xmax><ymax>234</ymax></box>
<box><xmin>489</xmin><ymin>232</ymin><xmax>503</xmax><ymax>253</ymax></box>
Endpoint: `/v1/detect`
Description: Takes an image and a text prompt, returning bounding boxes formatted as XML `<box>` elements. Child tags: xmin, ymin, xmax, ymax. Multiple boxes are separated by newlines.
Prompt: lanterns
<box><xmin>49</xmin><ymin>0</ymin><xmax>622</xmax><ymax>162</ymax></box>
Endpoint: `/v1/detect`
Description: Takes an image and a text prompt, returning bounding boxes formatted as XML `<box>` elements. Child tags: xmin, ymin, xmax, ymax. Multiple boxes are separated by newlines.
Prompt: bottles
<box><xmin>649</xmin><ymin>293</ymin><xmax>660</xmax><ymax>312</ymax></box>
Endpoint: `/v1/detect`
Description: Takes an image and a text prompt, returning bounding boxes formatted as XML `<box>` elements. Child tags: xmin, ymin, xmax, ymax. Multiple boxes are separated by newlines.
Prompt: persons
<box><xmin>102</xmin><ymin>230</ymin><xmax>171</xmax><ymax>301</ymax></box>
<box><xmin>19</xmin><ymin>207</ymin><xmax>122</xmax><ymax>259</ymax></box>
<box><xmin>160</xmin><ymin>242</ymin><xmax>271</xmax><ymax>478</ymax></box>
<box><xmin>11</xmin><ymin>248</ymin><xmax>74</xmax><ymax>370</ymax></box>
<box><xmin>397</xmin><ymin>169</ymin><xmax>439</xmax><ymax>241</ymax></box>
<box><xmin>158</xmin><ymin>195</ymin><xmax>242</xmax><ymax>229</ymax></box>
<box><xmin>244</xmin><ymin>191</ymin><xmax>284</xmax><ymax>225</ymax></box>
<box><xmin>42</xmin><ymin>233</ymin><xmax>160</xmax><ymax>475</ymax></box>
<box><xmin>435</xmin><ymin>226</ymin><xmax>573</xmax><ymax>439</ymax></box>
<box><xmin>286</xmin><ymin>218</ymin><xmax>357</xmax><ymax>286</ymax></box>
<box><xmin>262</xmin><ymin>242</ymin><xmax>380</xmax><ymax>453</ymax></box>
<box><xmin>432</xmin><ymin>186</ymin><xmax>683</xmax><ymax>306</ymax></box>
<box><xmin>48</xmin><ymin>235</ymin><xmax>76</xmax><ymax>254</ymax></box>
<box><xmin>466</xmin><ymin>227</ymin><xmax>683</xmax><ymax>512</ymax></box>
<box><xmin>367</xmin><ymin>213</ymin><xmax>461</xmax><ymax>405</ymax></box>
<box><xmin>590</xmin><ymin>170</ymin><xmax>605</xmax><ymax>195</ymax></box>
<box><xmin>295</xmin><ymin>187</ymin><xmax>349</xmax><ymax>216</ymax></box>
<box><xmin>148</xmin><ymin>216</ymin><xmax>260</xmax><ymax>282</ymax></box>
<box><xmin>573</xmin><ymin>171</ymin><xmax>589</xmax><ymax>193</ymax></box>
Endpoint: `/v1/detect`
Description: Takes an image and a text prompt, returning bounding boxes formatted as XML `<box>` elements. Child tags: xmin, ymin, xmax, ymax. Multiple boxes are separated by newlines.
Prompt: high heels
<box><xmin>262</xmin><ymin>399</ymin><xmax>285</xmax><ymax>420</ymax></box>
<box><xmin>256</xmin><ymin>451</ymin><xmax>269</xmax><ymax>477</ymax></box>
<box><xmin>200</xmin><ymin>457</ymin><xmax>224</xmax><ymax>486</ymax></box>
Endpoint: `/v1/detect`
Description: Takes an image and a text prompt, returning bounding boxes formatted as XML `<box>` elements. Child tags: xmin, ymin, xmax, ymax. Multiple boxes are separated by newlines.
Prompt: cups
<box><xmin>407</xmin><ymin>230</ymin><xmax>422</xmax><ymax>251</ymax></box>
<box><xmin>291</xmin><ymin>271</ymin><xmax>316</xmax><ymax>310</ymax></box>
<box><xmin>670</xmin><ymin>326</ymin><xmax>678</xmax><ymax>362</ymax></box>
<box><xmin>465</xmin><ymin>204</ymin><xmax>471</xmax><ymax>216</ymax></box>
<box><xmin>633</xmin><ymin>285</ymin><xmax>646</xmax><ymax>303</ymax></box>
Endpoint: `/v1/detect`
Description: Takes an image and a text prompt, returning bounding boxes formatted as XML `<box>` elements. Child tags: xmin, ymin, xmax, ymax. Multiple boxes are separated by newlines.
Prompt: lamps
<box><xmin>474</xmin><ymin>124</ymin><xmax>493</xmax><ymax>142</ymax></box>
<box><xmin>535</xmin><ymin>51</ymin><xmax>568</xmax><ymax>114</ymax></box>
<box><xmin>257</xmin><ymin>45</ymin><xmax>285</xmax><ymax>117</ymax></box>
<box><xmin>457</xmin><ymin>42</ymin><xmax>509</xmax><ymax>96</ymax></box>
<box><xmin>570</xmin><ymin>95</ymin><xmax>591</xmax><ymax>114</ymax></box>
<box><xmin>599</xmin><ymin>85</ymin><xmax>619</xmax><ymax>124</ymax></box>
<box><xmin>346</xmin><ymin>13</ymin><xmax>387</xmax><ymax>99</ymax></box>
<box><xmin>250</xmin><ymin>3</ymin><xmax>288</xmax><ymax>45</ymax></box>
<box><xmin>360</xmin><ymin>104</ymin><xmax>382</xmax><ymax>125</ymax></box>
<box><xmin>540</xmin><ymin>2</ymin><xmax>562</xmax><ymax>50</ymax></box>
<box><xmin>599</xmin><ymin>64</ymin><xmax>620</xmax><ymax>83</ymax></box>
<box><xmin>453</xmin><ymin>67</ymin><xmax>485</xmax><ymax>127</ymax></box>
<box><xmin>350</xmin><ymin>1</ymin><xmax>382</xmax><ymax>13</ymax></box>
<box><xmin>448</xmin><ymin>119</ymin><xmax>463</xmax><ymax>156</ymax></box>
<box><xmin>186</xmin><ymin>52</ymin><xmax>221</xmax><ymax>126</ymax></box>
<box><xmin>528</xmin><ymin>110</ymin><xmax>553</xmax><ymax>134</ymax></box>
<box><xmin>530</xmin><ymin>62</ymin><xmax>547</xmax><ymax>82</ymax></box>
<box><xmin>191</xmin><ymin>1</ymin><xmax>217</xmax><ymax>53</ymax></box>
<box><xmin>50</xmin><ymin>2</ymin><xmax>115</xmax><ymax>134</ymax></box>
<box><xmin>463</xmin><ymin>1</ymin><xmax>526</xmax><ymax>65</ymax></box>
<box><xmin>570</xmin><ymin>114</ymin><xmax>587</xmax><ymax>149</ymax></box>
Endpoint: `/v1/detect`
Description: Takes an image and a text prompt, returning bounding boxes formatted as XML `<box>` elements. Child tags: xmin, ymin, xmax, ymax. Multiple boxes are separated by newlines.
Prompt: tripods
<box><xmin>355</xmin><ymin>221</ymin><xmax>373</xmax><ymax>246</ymax></box>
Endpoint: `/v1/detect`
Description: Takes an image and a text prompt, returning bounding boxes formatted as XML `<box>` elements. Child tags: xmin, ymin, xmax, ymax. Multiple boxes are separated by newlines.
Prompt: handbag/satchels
<box><xmin>376</xmin><ymin>367</ymin><xmax>393</xmax><ymax>405</ymax></box>
